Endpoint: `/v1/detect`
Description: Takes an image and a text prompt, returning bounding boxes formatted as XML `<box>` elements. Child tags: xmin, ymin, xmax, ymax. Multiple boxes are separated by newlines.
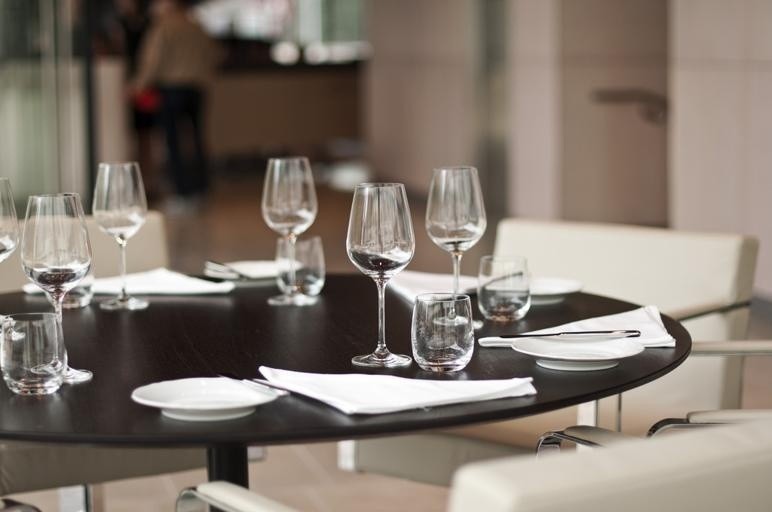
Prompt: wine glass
<box><xmin>346</xmin><ymin>182</ymin><xmax>416</xmax><ymax>368</ymax></box>
<box><xmin>261</xmin><ymin>156</ymin><xmax>319</xmax><ymax>307</ymax></box>
<box><xmin>424</xmin><ymin>166</ymin><xmax>488</xmax><ymax>295</ymax></box>
<box><xmin>0</xmin><ymin>160</ymin><xmax>148</xmax><ymax>384</ymax></box>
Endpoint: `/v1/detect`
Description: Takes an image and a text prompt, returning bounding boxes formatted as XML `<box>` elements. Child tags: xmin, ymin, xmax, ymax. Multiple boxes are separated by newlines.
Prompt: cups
<box><xmin>275</xmin><ymin>234</ymin><xmax>326</xmax><ymax>296</ymax></box>
<box><xmin>411</xmin><ymin>293</ymin><xmax>475</xmax><ymax>373</ymax></box>
<box><xmin>477</xmin><ymin>254</ymin><xmax>531</xmax><ymax>321</ymax></box>
<box><xmin>0</xmin><ymin>313</ymin><xmax>62</xmax><ymax>397</ymax></box>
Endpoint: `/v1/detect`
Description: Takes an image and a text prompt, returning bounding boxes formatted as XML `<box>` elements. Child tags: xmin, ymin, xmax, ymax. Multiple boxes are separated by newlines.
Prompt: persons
<box><xmin>126</xmin><ymin>1</ymin><xmax>222</xmax><ymax>219</ymax></box>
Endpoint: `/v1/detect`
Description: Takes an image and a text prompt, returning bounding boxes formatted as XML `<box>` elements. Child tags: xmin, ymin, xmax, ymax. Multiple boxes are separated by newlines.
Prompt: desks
<box><xmin>0</xmin><ymin>272</ymin><xmax>692</xmax><ymax>511</ymax></box>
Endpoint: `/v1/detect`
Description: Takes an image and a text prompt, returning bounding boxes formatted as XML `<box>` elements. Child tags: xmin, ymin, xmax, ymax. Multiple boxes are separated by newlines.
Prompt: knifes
<box><xmin>499</xmin><ymin>330</ymin><xmax>641</xmax><ymax>339</ymax></box>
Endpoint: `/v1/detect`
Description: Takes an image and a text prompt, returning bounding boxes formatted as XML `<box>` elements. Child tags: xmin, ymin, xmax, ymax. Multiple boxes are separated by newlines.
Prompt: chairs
<box><xmin>479</xmin><ymin>218</ymin><xmax>758</xmax><ymax>432</ymax></box>
<box><xmin>1</xmin><ymin>212</ymin><xmax>166</xmax><ymax>511</ymax></box>
<box><xmin>645</xmin><ymin>339</ymin><xmax>772</xmax><ymax>429</ymax></box>
<box><xmin>174</xmin><ymin>420</ymin><xmax>771</xmax><ymax>512</ymax></box>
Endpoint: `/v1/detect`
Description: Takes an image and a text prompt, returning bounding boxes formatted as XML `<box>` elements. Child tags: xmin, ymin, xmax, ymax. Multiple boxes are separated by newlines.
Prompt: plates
<box><xmin>510</xmin><ymin>337</ymin><xmax>645</xmax><ymax>371</ymax></box>
<box><xmin>531</xmin><ymin>278</ymin><xmax>583</xmax><ymax>306</ymax></box>
<box><xmin>131</xmin><ymin>377</ymin><xmax>279</xmax><ymax>422</ymax></box>
<box><xmin>204</xmin><ymin>260</ymin><xmax>278</xmax><ymax>289</ymax></box>
